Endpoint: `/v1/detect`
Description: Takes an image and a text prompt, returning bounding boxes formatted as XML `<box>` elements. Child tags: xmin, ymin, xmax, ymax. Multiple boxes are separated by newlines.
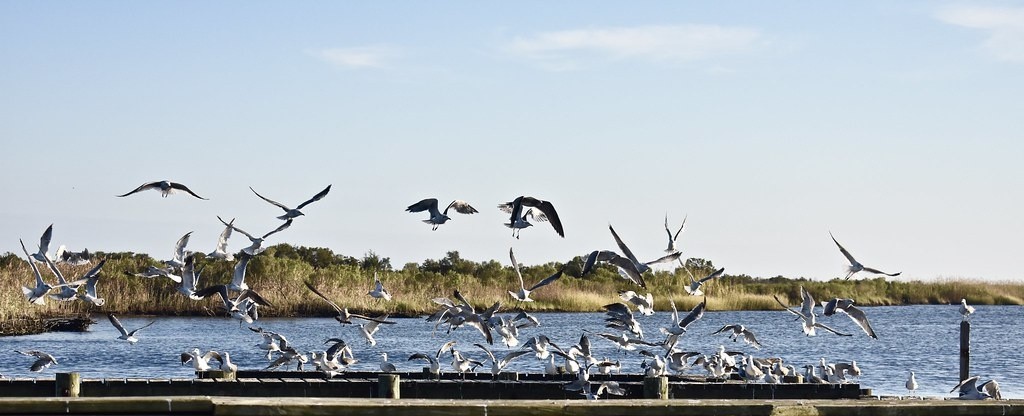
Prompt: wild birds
<box><xmin>115</xmin><ymin>180</ymin><xmax>211</xmax><ymax>201</ymax></box>
<box><xmin>959</xmin><ymin>298</ymin><xmax>976</xmax><ymax>321</ymax></box>
<box><xmin>678</xmin><ymin>258</ymin><xmax>726</xmax><ymax>297</ymax></box>
<box><xmin>664</xmin><ymin>213</ymin><xmax>688</xmax><ymax>255</ymax></box>
<box><xmin>504</xmin><ymin>196</ymin><xmax>549</xmax><ymax>240</ymax></box>
<box><xmin>405</xmin><ymin>197</ymin><xmax>479</xmax><ymax>231</ymax></box>
<box><xmin>905</xmin><ymin>371</ymin><xmax>919</xmax><ymax>395</ymax></box>
<box><xmin>105</xmin><ymin>313</ymin><xmax>155</xmax><ymax>346</ymax></box>
<box><xmin>18</xmin><ymin>214</ymin><xmax>863</xmax><ymax>384</ymax></box>
<box><xmin>712</xmin><ymin>323</ymin><xmax>763</xmax><ymax>350</ymax></box>
<box><xmin>578</xmin><ymin>250</ymin><xmax>648</xmax><ymax>290</ymax></box>
<box><xmin>828</xmin><ymin>229</ymin><xmax>904</xmax><ymax>281</ymax></box>
<box><xmin>608</xmin><ymin>225</ymin><xmax>683</xmax><ymax>285</ymax></box>
<box><xmin>497</xmin><ymin>195</ymin><xmax>566</xmax><ymax>238</ymax></box>
<box><xmin>249</xmin><ymin>183</ymin><xmax>333</xmax><ymax>226</ymax></box>
<box><xmin>14</xmin><ymin>349</ymin><xmax>59</xmax><ymax>372</ymax></box>
<box><xmin>773</xmin><ymin>285</ymin><xmax>854</xmax><ymax>338</ymax></box>
<box><xmin>949</xmin><ymin>375</ymin><xmax>1002</xmax><ymax>401</ymax></box>
<box><xmin>507</xmin><ymin>247</ymin><xmax>563</xmax><ymax>311</ymax></box>
<box><xmin>818</xmin><ymin>298</ymin><xmax>879</xmax><ymax>340</ymax></box>
